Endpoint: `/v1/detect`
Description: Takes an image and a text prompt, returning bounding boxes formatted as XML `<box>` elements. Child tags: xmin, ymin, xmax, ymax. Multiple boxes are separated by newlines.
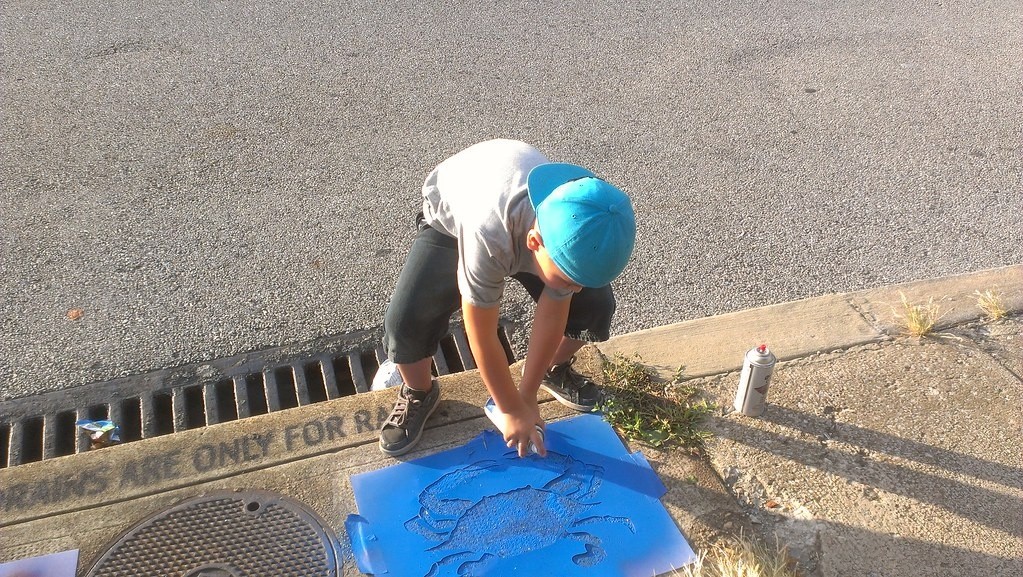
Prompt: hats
<box><xmin>526</xmin><ymin>162</ymin><xmax>636</xmax><ymax>289</ymax></box>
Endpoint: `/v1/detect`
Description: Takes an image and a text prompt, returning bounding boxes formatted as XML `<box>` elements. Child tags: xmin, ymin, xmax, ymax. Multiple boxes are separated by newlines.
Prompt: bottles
<box><xmin>484</xmin><ymin>396</ymin><xmax>545</xmax><ymax>455</ymax></box>
<box><xmin>734</xmin><ymin>345</ymin><xmax>776</xmax><ymax>416</ymax></box>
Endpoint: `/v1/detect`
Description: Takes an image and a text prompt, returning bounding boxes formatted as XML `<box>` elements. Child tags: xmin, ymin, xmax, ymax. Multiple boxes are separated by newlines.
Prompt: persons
<box><xmin>377</xmin><ymin>138</ymin><xmax>636</xmax><ymax>457</ymax></box>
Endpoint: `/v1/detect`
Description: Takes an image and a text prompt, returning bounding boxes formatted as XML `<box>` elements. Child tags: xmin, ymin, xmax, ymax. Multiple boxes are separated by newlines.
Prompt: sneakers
<box><xmin>521</xmin><ymin>356</ymin><xmax>604</xmax><ymax>412</ymax></box>
<box><xmin>379</xmin><ymin>374</ymin><xmax>441</xmax><ymax>456</ymax></box>
